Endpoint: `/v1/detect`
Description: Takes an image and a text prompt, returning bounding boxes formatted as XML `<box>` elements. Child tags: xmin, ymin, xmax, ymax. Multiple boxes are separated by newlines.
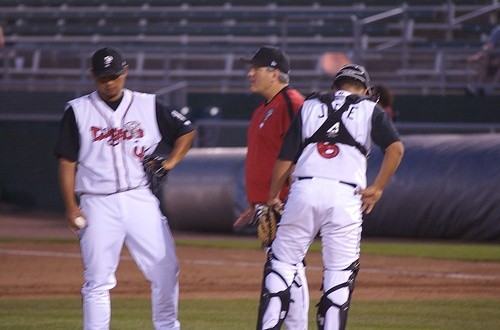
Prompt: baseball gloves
<box><xmin>253</xmin><ymin>203</ymin><xmax>276</xmax><ymax>247</ymax></box>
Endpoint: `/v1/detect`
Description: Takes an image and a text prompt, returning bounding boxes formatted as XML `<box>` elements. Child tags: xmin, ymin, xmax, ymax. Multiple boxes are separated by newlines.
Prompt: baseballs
<box><xmin>72</xmin><ymin>217</ymin><xmax>86</xmax><ymax>229</ymax></box>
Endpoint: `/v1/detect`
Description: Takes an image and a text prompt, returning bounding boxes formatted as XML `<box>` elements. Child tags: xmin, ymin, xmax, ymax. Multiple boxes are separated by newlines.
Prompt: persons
<box><xmin>54</xmin><ymin>47</ymin><xmax>195</xmax><ymax>330</ymax></box>
<box><xmin>470</xmin><ymin>9</ymin><xmax>500</xmax><ymax>95</ymax></box>
<box><xmin>255</xmin><ymin>64</ymin><xmax>405</xmax><ymax>330</ymax></box>
<box><xmin>238</xmin><ymin>46</ymin><xmax>310</xmax><ymax>330</ymax></box>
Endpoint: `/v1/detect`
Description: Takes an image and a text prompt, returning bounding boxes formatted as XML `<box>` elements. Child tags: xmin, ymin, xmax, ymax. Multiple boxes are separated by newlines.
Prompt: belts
<box><xmin>298</xmin><ymin>176</ymin><xmax>356</xmax><ymax>187</ymax></box>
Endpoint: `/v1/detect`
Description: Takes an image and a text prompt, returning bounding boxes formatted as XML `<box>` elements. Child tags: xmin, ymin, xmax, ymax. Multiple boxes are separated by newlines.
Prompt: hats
<box><xmin>240</xmin><ymin>45</ymin><xmax>290</xmax><ymax>73</ymax></box>
<box><xmin>332</xmin><ymin>64</ymin><xmax>373</xmax><ymax>96</ymax></box>
<box><xmin>90</xmin><ymin>47</ymin><xmax>126</xmax><ymax>79</ymax></box>
